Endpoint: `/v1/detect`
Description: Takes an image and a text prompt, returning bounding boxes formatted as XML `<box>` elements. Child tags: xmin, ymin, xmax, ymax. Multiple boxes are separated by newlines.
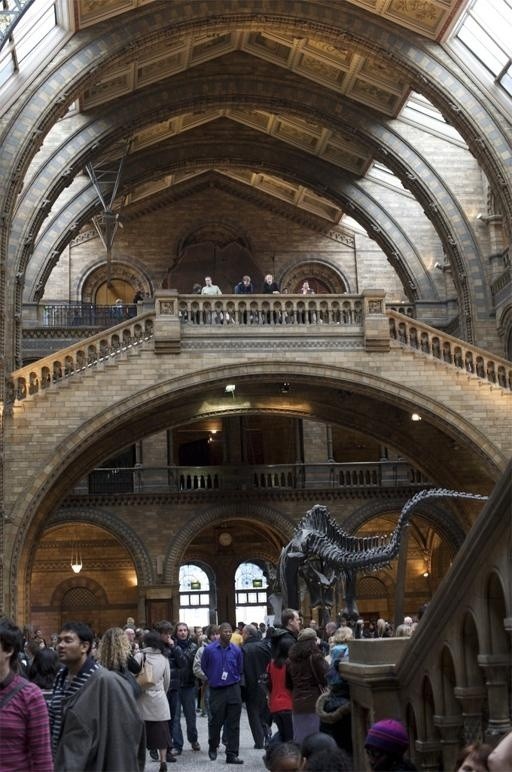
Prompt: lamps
<box><xmin>70</xmin><ymin>538</ymin><xmax>84</xmax><ymax>574</ymax></box>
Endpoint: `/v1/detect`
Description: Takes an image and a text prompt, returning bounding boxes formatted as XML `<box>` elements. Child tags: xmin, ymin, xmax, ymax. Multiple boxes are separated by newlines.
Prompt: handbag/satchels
<box><xmin>134</xmin><ymin>661</ymin><xmax>156</xmax><ymax>688</ymax></box>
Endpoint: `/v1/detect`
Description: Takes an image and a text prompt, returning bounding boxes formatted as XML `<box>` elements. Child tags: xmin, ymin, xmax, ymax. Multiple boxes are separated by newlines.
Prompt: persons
<box><xmin>132</xmin><ymin>285</ymin><xmax>144</xmax><ymax>304</ymax></box>
<box><xmin>186</xmin><ymin>282</ymin><xmax>203</xmax><ymax>322</ymax></box>
<box><xmin>261</xmin><ymin>273</ymin><xmax>281</xmax><ymax>325</ymax></box>
<box><xmin>295</xmin><ymin>281</ymin><xmax>316</xmax><ymax>327</ymax></box>
<box><xmin>201</xmin><ymin>275</ymin><xmax>224</xmax><ymax>325</ymax></box>
<box><xmin>232</xmin><ymin>275</ymin><xmax>253</xmax><ymax>325</ymax></box>
<box><xmin>109</xmin><ymin>298</ymin><xmax>126</xmax><ymax>321</ymax></box>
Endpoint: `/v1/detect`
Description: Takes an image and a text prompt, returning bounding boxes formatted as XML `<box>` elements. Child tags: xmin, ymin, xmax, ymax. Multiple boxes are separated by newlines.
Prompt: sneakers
<box><xmin>149</xmin><ymin>748</ymin><xmax>159</xmax><ymax>760</ymax></box>
<box><xmin>167</xmin><ymin>744</ymin><xmax>183</xmax><ymax>762</ymax></box>
<box><xmin>190</xmin><ymin>741</ymin><xmax>201</xmax><ymax>751</ymax></box>
<box><xmin>264</xmin><ymin>734</ymin><xmax>272</xmax><ymax>748</ymax></box>
<box><xmin>158</xmin><ymin>762</ymin><xmax>167</xmax><ymax>772</ymax></box>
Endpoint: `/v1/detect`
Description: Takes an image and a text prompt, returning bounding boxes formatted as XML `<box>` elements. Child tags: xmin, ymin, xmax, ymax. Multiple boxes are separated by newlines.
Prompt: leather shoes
<box><xmin>208</xmin><ymin>747</ymin><xmax>218</xmax><ymax>762</ymax></box>
<box><xmin>225</xmin><ymin>755</ymin><xmax>245</xmax><ymax>765</ymax></box>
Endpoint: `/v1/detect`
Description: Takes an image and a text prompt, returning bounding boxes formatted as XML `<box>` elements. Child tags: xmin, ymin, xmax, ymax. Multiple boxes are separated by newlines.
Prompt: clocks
<box><xmin>217</xmin><ymin>530</ymin><xmax>234</xmax><ymax>547</ymax></box>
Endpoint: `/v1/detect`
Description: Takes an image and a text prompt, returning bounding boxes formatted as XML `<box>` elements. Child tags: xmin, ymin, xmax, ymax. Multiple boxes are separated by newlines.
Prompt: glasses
<box><xmin>365</xmin><ymin>749</ymin><xmax>385</xmax><ymax>759</ymax></box>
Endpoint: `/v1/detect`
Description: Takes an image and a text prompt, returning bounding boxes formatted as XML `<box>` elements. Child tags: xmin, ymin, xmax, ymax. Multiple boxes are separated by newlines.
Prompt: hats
<box><xmin>363</xmin><ymin>718</ymin><xmax>411</xmax><ymax>759</ymax></box>
<box><xmin>296</xmin><ymin>627</ymin><xmax>318</xmax><ymax>642</ymax></box>
